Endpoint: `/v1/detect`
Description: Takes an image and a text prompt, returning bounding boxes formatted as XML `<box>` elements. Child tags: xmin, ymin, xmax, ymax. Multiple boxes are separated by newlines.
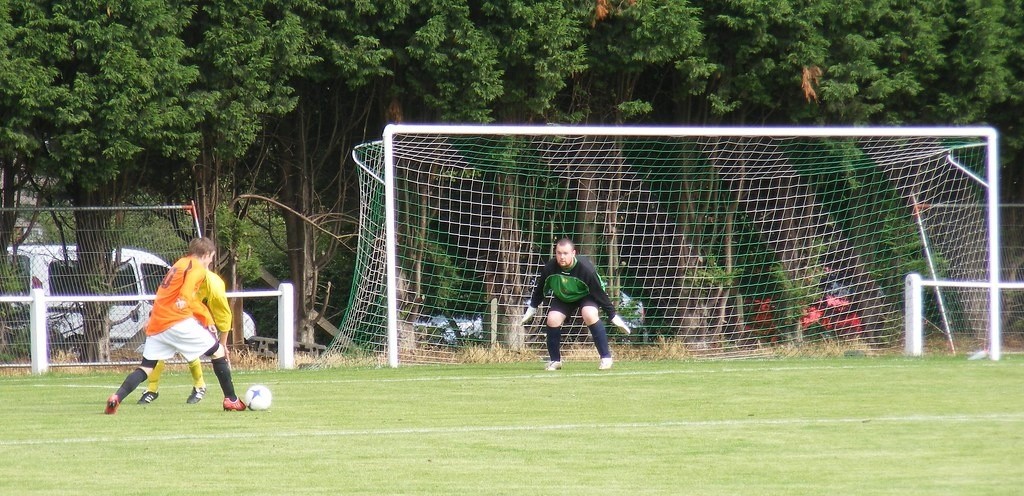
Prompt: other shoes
<box><xmin>546</xmin><ymin>359</ymin><xmax>563</xmax><ymax>371</ymax></box>
<box><xmin>599</xmin><ymin>356</ymin><xmax>612</xmax><ymax>369</ymax></box>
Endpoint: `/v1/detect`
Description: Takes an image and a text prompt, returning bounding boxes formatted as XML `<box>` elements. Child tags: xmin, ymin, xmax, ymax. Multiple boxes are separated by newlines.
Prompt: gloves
<box><xmin>520</xmin><ymin>305</ymin><xmax>535</xmax><ymax>326</ymax></box>
<box><xmin>612</xmin><ymin>314</ymin><xmax>631</xmax><ymax>336</ymax></box>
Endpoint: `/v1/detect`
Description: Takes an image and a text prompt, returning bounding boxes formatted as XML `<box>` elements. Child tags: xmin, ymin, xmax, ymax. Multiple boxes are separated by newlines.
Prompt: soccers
<box><xmin>244</xmin><ymin>384</ymin><xmax>273</xmax><ymax>410</ymax></box>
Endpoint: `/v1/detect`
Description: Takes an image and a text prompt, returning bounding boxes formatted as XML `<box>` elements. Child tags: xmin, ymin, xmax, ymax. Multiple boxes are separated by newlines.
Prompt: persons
<box><xmin>522</xmin><ymin>240</ymin><xmax>631</xmax><ymax>370</ymax></box>
<box><xmin>138</xmin><ymin>301</ymin><xmax>219</xmax><ymax>406</ymax></box>
<box><xmin>105</xmin><ymin>237</ymin><xmax>245</xmax><ymax>414</ymax></box>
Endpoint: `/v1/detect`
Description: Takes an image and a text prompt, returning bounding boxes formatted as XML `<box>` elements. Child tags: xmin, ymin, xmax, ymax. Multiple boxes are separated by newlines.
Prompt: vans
<box><xmin>4</xmin><ymin>246</ymin><xmax>256</xmax><ymax>356</ymax></box>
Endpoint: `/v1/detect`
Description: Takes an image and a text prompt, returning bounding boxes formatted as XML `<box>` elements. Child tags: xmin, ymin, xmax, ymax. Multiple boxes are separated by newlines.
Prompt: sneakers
<box><xmin>223</xmin><ymin>397</ymin><xmax>246</xmax><ymax>412</ymax></box>
<box><xmin>187</xmin><ymin>384</ymin><xmax>206</xmax><ymax>404</ymax></box>
<box><xmin>137</xmin><ymin>387</ymin><xmax>159</xmax><ymax>405</ymax></box>
<box><xmin>105</xmin><ymin>394</ymin><xmax>120</xmax><ymax>414</ymax></box>
